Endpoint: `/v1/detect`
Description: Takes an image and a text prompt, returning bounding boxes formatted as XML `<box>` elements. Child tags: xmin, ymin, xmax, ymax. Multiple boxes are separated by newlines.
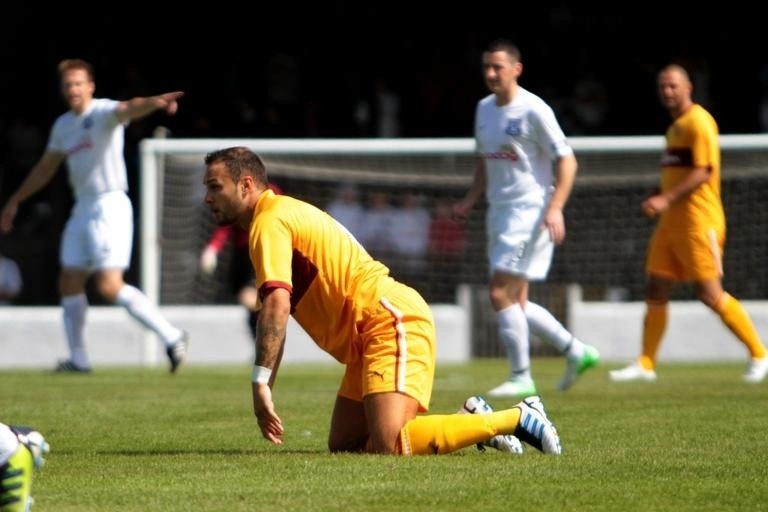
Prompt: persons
<box><xmin>428</xmin><ymin>202</ymin><xmax>461</xmax><ymax>300</ymax></box>
<box><xmin>0</xmin><ymin>422</ymin><xmax>51</xmax><ymax>512</ymax></box>
<box><xmin>0</xmin><ymin>257</ymin><xmax>21</xmax><ymax>307</ymax></box>
<box><xmin>358</xmin><ymin>190</ymin><xmax>397</xmax><ymax>277</ymax></box>
<box><xmin>0</xmin><ymin>61</ymin><xmax>190</xmax><ymax>374</ymax></box>
<box><xmin>199</xmin><ymin>181</ymin><xmax>283</xmax><ymax>312</ymax></box>
<box><xmin>323</xmin><ymin>184</ymin><xmax>361</xmax><ymax>243</ymax></box>
<box><xmin>457</xmin><ymin>41</ymin><xmax>601</xmax><ymax>398</ymax></box>
<box><xmin>203</xmin><ymin>148</ymin><xmax>562</xmax><ymax>455</ymax></box>
<box><xmin>608</xmin><ymin>64</ymin><xmax>767</xmax><ymax>380</ymax></box>
<box><xmin>386</xmin><ymin>188</ymin><xmax>429</xmax><ymax>284</ymax></box>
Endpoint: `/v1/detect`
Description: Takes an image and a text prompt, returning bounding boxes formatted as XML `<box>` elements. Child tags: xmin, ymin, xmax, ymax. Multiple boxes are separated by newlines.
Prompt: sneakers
<box><xmin>459</xmin><ymin>395</ymin><xmax>523</xmax><ymax>454</ymax></box>
<box><xmin>55</xmin><ymin>360</ymin><xmax>93</xmax><ymax>373</ymax></box>
<box><xmin>166</xmin><ymin>330</ymin><xmax>192</xmax><ymax>375</ymax></box>
<box><xmin>485</xmin><ymin>375</ymin><xmax>536</xmax><ymax>398</ymax></box>
<box><xmin>740</xmin><ymin>351</ymin><xmax>768</xmax><ymax>383</ymax></box>
<box><xmin>557</xmin><ymin>344</ymin><xmax>599</xmax><ymax>391</ymax></box>
<box><xmin>511</xmin><ymin>395</ymin><xmax>562</xmax><ymax>455</ymax></box>
<box><xmin>608</xmin><ymin>363</ymin><xmax>657</xmax><ymax>382</ymax></box>
